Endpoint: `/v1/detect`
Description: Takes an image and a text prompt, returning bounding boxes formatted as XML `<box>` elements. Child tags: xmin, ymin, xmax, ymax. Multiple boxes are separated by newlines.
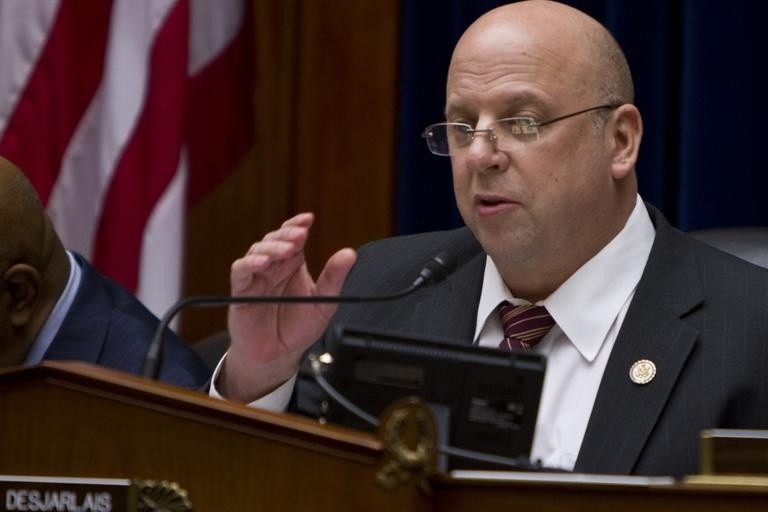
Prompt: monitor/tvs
<box><xmin>317</xmin><ymin>325</ymin><xmax>547</xmax><ymax>470</ymax></box>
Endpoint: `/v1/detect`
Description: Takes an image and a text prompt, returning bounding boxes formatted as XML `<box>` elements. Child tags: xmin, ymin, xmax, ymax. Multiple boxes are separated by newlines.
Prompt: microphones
<box><xmin>140</xmin><ymin>253</ymin><xmax>456</xmax><ymax>382</ymax></box>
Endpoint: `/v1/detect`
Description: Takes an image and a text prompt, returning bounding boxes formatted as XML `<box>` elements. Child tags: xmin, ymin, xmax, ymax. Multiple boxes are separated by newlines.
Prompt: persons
<box><xmin>198</xmin><ymin>2</ymin><xmax>768</xmax><ymax>474</ymax></box>
<box><xmin>1</xmin><ymin>155</ymin><xmax>212</xmax><ymax>397</ymax></box>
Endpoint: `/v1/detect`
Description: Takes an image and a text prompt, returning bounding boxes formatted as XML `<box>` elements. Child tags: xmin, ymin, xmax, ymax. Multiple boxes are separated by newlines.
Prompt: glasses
<box><xmin>420</xmin><ymin>105</ymin><xmax>619</xmax><ymax>157</ymax></box>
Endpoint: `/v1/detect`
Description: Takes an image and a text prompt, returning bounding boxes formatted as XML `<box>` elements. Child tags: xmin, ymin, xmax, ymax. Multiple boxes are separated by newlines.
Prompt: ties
<box><xmin>494</xmin><ymin>299</ymin><xmax>554</xmax><ymax>355</ymax></box>
